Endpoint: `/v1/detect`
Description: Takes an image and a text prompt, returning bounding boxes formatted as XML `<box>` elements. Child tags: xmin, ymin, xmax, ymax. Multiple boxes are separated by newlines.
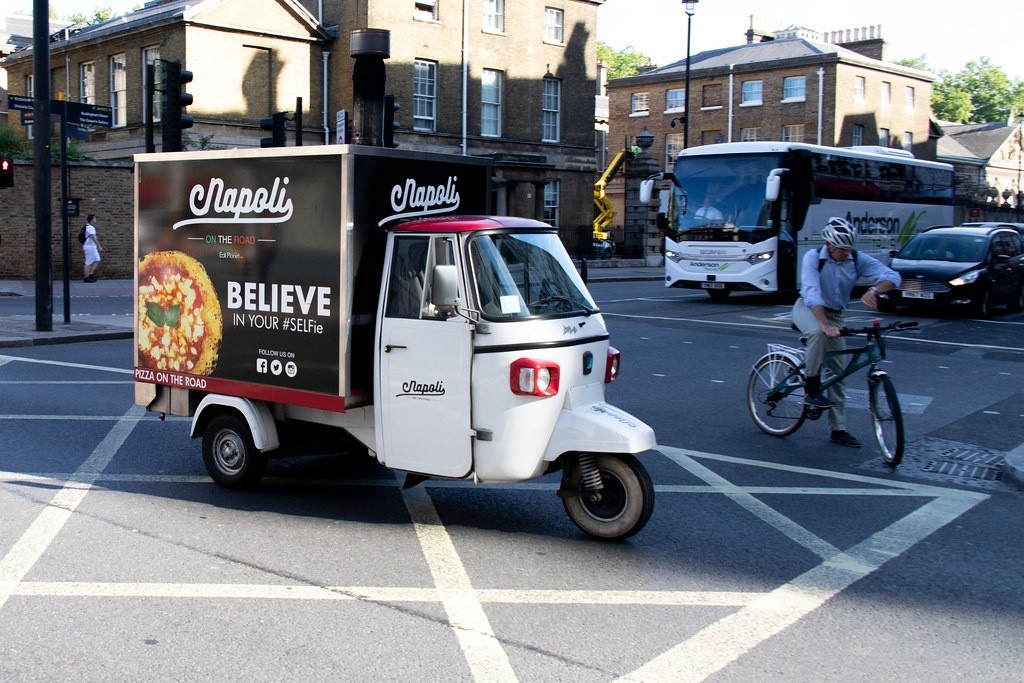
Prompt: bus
<box><xmin>639</xmin><ymin>141</ymin><xmax>955</xmax><ymax>306</ymax></box>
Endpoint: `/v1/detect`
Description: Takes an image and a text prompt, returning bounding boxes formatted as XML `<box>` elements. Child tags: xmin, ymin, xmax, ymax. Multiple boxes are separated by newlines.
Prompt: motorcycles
<box><xmin>131</xmin><ymin>144</ymin><xmax>659</xmax><ymax>543</ymax></box>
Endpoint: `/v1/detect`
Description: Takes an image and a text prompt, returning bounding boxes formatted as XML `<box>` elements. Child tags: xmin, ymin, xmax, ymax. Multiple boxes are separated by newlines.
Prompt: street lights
<box><xmin>679</xmin><ymin>0</ymin><xmax>698</xmax><ymax>150</ymax></box>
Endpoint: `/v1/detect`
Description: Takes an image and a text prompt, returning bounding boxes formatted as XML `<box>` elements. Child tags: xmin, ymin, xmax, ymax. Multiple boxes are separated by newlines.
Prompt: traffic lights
<box><xmin>0</xmin><ymin>156</ymin><xmax>15</xmax><ymax>190</ymax></box>
<box><xmin>160</xmin><ymin>60</ymin><xmax>195</xmax><ymax>152</ymax></box>
<box><xmin>259</xmin><ymin>110</ymin><xmax>288</xmax><ymax>148</ymax></box>
<box><xmin>383</xmin><ymin>94</ymin><xmax>402</xmax><ymax>149</ymax></box>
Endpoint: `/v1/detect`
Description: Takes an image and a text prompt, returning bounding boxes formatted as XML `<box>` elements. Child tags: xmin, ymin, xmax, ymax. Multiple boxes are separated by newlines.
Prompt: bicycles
<box><xmin>745</xmin><ymin>320</ymin><xmax>922</xmax><ymax>466</ymax></box>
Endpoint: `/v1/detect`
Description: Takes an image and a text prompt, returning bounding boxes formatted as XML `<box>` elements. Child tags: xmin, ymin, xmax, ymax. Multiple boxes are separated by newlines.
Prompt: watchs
<box><xmin>868</xmin><ymin>287</ymin><xmax>878</xmax><ymax>294</ymax></box>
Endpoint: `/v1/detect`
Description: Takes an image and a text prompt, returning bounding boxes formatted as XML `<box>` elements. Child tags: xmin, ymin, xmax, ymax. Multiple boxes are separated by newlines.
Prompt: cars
<box><xmin>874</xmin><ymin>223</ymin><xmax>1024</xmax><ymax>318</ymax></box>
<box><xmin>593</xmin><ymin>236</ymin><xmax>616</xmax><ymax>260</ymax></box>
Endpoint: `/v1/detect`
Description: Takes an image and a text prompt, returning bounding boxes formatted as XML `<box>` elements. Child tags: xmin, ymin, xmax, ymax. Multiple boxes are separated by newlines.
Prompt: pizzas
<box><xmin>136</xmin><ymin>250</ymin><xmax>223</xmax><ymax>376</ymax></box>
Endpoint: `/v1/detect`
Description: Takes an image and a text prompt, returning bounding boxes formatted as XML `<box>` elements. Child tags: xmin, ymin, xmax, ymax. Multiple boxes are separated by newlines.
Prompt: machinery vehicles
<box><xmin>592</xmin><ymin>147</ymin><xmax>630</xmax><ymax>242</ymax></box>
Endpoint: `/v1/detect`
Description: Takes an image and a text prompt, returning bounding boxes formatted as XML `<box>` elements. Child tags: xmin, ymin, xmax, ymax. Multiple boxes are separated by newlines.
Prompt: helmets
<box><xmin>822</xmin><ymin>216</ymin><xmax>859</xmax><ymax>251</ymax></box>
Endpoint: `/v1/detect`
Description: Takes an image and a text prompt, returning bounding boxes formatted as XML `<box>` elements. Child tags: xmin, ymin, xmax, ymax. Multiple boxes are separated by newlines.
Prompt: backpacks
<box><xmin>78</xmin><ymin>224</ymin><xmax>93</xmax><ymax>244</ymax></box>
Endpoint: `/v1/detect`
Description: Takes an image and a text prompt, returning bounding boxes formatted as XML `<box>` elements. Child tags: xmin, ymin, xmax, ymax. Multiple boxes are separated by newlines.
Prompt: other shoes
<box><xmin>84</xmin><ymin>277</ymin><xmax>96</xmax><ymax>283</ymax></box>
<box><xmin>829</xmin><ymin>431</ymin><xmax>862</xmax><ymax>447</ymax></box>
<box><xmin>802</xmin><ymin>393</ymin><xmax>838</xmax><ymax>408</ymax></box>
<box><xmin>89</xmin><ymin>276</ymin><xmax>97</xmax><ymax>281</ymax></box>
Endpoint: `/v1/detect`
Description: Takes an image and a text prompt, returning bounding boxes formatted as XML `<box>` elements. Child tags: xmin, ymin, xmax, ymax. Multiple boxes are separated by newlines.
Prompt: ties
<box><xmin>702</xmin><ymin>209</ymin><xmax>709</xmax><ymax>217</ymax></box>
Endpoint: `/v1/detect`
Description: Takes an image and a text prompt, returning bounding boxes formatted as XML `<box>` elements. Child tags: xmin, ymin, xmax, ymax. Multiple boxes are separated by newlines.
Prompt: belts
<box><xmin>824</xmin><ymin>306</ymin><xmax>842</xmax><ymax>315</ymax></box>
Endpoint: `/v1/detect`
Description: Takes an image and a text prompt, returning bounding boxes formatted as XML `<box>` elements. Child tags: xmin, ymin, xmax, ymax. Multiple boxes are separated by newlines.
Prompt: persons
<box><xmin>693</xmin><ymin>198</ymin><xmax>724</xmax><ymax>220</ymax></box>
<box><xmin>82</xmin><ymin>214</ymin><xmax>103</xmax><ymax>283</ymax></box>
<box><xmin>791</xmin><ymin>216</ymin><xmax>901</xmax><ymax>445</ymax></box>
<box><xmin>925</xmin><ymin>241</ymin><xmax>954</xmax><ymax>258</ymax></box>
